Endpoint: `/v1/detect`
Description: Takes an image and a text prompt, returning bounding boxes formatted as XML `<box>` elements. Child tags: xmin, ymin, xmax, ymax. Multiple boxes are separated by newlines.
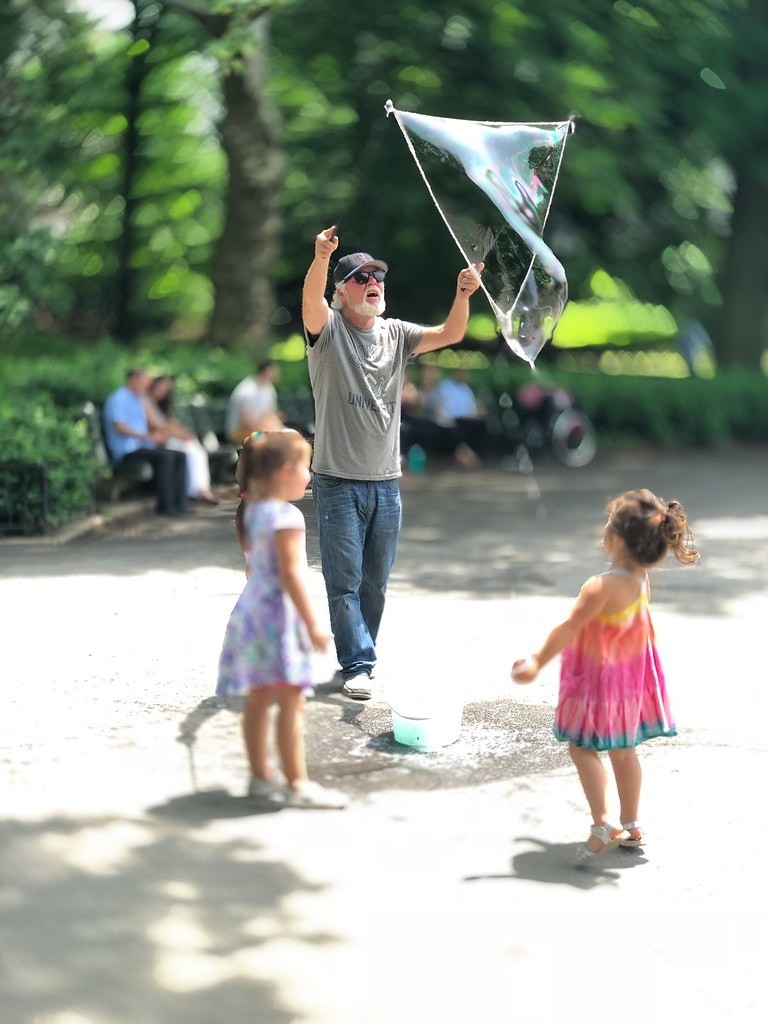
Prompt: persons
<box><xmin>511</xmin><ymin>488</ymin><xmax>701</xmax><ymax>871</ymax></box>
<box><xmin>107</xmin><ymin>355</ymin><xmax>594</xmax><ymax>517</ymax></box>
<box><xmin>216</xmin><ymin>427</ymin><xmax>348</xmax><ymax>809</ymax></box>
<box><xmin>303</xmin><ymin>225</ymin><xmax>486</xmax><ymax>701</ymax></box>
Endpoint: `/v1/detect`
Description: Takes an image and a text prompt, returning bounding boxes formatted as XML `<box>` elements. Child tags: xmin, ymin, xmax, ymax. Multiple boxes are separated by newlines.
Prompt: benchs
<box><xmin>101</xmin><ymin>405</ymin><xmax>241</xmax><ymax>501</ymax></box>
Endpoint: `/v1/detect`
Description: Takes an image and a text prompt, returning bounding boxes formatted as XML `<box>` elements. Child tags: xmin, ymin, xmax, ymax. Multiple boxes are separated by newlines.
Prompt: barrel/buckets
<box><xmin>380</xmin><ymin>677</ymin><xmax>464</xmax><ymax>749</ymax></box>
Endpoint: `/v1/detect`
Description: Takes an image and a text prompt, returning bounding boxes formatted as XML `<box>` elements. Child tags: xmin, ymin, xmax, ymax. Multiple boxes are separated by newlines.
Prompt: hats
<box><xmin>333</xmin><ymin>252</ymin><xmax>389</xmax><ymax>286</ymax></box>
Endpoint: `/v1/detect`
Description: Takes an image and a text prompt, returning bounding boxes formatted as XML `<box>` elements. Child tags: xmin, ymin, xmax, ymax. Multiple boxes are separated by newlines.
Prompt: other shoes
<box><xmin>370</xmin><ymin>667</ymin><xmax>377</xmax><ymax>679</ymax></box>
<box><xmin>248</xmin><ymin>771</ymin><xmax>288</xmax><ymax>798</ymax></box>
<box><xmin>191</xmin><ymin>492</ymin><xmax>219</xmax><ymax>505</ymax></box>
<box><xmin>282</xmin><ymin>778</ymin><xmax>350</xmax><ymax>809</ymax></box>
<box><xmin>343</xmin><ymin>671</ymin><xmax>373</xmax><ymax>699</ymax></box>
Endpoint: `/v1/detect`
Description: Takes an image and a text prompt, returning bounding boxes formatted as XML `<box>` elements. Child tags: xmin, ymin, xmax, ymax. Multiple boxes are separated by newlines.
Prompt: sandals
<box><xmin>575</xmin><ymin>820</ymin><xmax>633</xmax><ymax>865</ymax></box>
<box><xmin>620</xmin><ymin>820</ymin><xmax>648</xmax><ymax>847</ymax></box>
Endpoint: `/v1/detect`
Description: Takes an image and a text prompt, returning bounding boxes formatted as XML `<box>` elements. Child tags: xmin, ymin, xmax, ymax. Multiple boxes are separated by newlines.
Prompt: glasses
<box><xmin>352</xmin><ymin>270</ymin><xmax>386</xmax><ymax>284</ymax></box>
